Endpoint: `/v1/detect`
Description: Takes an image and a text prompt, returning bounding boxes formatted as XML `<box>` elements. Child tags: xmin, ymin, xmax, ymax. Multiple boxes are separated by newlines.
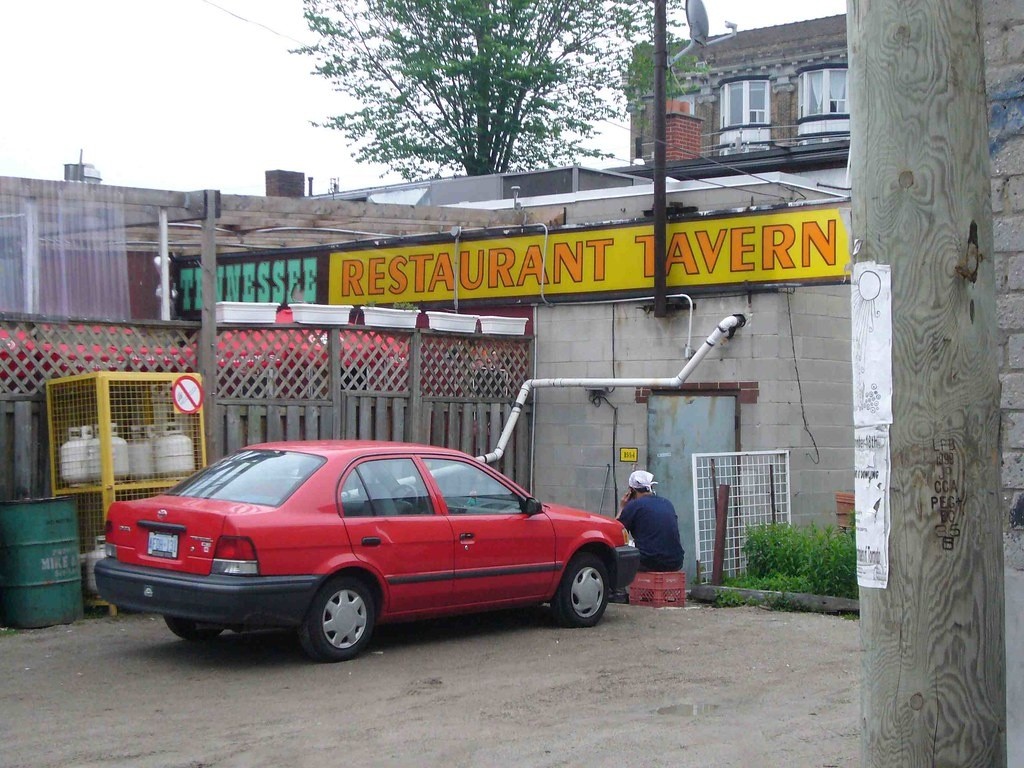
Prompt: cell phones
<box><xmin>627</xmin><ymin>488</ymin><xmax>636</xmax><ymax>501</ymax></box>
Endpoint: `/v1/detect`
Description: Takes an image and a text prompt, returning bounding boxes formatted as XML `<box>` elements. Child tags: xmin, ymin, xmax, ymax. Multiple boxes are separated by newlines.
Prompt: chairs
<box><xmin>362</xmin><ymin>483</ymin><xmax>399</xmax><ymax>516</ymax></box>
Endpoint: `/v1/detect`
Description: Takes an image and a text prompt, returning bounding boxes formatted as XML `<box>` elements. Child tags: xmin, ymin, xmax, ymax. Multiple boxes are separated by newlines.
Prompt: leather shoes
<box><xmin>608</xmin><ymin>588</ymin><xmax>629</xmax><ymax>604</ymax></box>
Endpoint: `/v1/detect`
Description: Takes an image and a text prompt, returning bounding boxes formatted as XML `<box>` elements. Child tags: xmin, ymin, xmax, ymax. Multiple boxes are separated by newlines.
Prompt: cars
<box><xmin>92</xmin><ymin>438</ymin><xmax>641</xmax><ymax>664</ymax></box>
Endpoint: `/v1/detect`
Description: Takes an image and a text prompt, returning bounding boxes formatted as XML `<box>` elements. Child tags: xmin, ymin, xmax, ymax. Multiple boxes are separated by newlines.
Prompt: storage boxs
<box><xmin>630</xmin><ymin>571</ymin><xmax>686</xmax><ymax>608</ymax></box>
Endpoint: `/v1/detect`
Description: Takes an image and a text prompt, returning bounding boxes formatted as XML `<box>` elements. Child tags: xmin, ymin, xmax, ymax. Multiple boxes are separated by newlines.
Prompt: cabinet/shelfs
<box><xmin>46</xmin><ymin>371</ymin><xmax>208</xmax><ymax>618</ymax></box>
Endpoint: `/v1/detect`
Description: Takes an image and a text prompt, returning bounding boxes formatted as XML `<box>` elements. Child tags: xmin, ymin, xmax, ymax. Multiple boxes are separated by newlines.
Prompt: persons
<box><xmin>609</xmin><ymin>470</ymin><xmax>685</xmax><ymax>604</ymax></box>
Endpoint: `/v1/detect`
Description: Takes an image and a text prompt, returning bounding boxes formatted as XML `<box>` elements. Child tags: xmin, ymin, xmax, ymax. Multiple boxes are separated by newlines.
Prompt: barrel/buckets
<box><xmin>0</xmin><ymin>496</ymin><xmax>84</xmax><ymax>628</ymax></box>
